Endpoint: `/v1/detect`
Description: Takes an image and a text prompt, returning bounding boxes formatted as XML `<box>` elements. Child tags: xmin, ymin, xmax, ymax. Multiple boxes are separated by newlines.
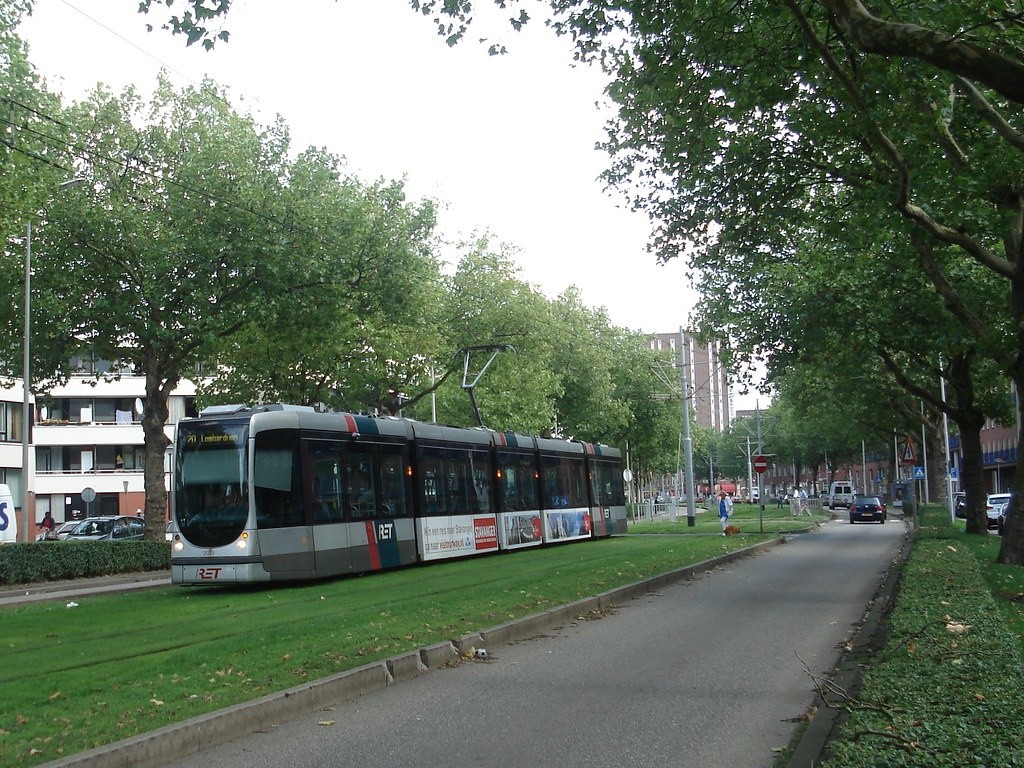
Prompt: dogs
<box><xmin>723</xmin><ymin>526</ymin><xmax>740</xmax><ymax>538</ymax></box>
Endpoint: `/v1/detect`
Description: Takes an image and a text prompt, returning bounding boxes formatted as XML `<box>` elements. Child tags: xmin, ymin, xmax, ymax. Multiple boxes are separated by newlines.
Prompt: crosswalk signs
<box><xmin>914</xmin><ymin>467</ymin><xmax>925</xmax><ymax>479</ymax></box>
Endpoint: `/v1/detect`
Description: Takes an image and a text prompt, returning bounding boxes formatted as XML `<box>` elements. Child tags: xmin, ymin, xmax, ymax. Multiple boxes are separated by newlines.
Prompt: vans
<box><xmin>829</xmin><ymin>480</ymin><xmax>854</xmax><ymax>509</ymax></box>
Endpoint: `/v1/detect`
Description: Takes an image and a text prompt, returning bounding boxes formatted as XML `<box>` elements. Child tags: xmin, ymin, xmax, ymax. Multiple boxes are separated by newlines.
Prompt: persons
<box><xmin>717</xmin><ymin>490</ymin><xmax>733</xmax><ymax>536</ymax></box>
<box><xmin>602</xmin><ymin>484</ymin><xmax>608</xmax><ymax>502</ymax></box>
<box><xmin>474</xmin><ymin>467</ymin><xmax>490</xmax><ymax>514</ymax></box>
<box><xmin>894</xmin><ymin>488</ymin><xmax>905</xmax><ymax>501</ymax></box>
<box><xmin>39</xmin><ymin>511</ymin><xmax>56</xmax><ymax>540</ymax></box>
<box><xmin>423</xmin><ymin>495</ymin><xmax>447</xmax><ymax>511</ymax></box>
<box><xmin>776</xmin><ymin>485</ymin><xmax>812</xmax><ymax>517</ymax></box>
<box><xmin>113</xmin><ymin>455</ymin><xmax>125</xmax><ymax>473</ymax></box>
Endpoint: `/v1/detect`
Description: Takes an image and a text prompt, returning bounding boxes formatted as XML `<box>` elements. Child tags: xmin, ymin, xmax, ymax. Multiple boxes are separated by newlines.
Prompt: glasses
<box><xmin>719</xmin><ymin>494</ymin><xmax>723</xmax><ymax>495</ymax></box>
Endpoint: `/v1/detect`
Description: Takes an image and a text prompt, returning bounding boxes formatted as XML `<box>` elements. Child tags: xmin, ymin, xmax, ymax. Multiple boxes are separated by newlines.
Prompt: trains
<box><xmin>170</xmin><ymin>402</ymin><xmax>627</xmax><ymax>591</ymax></box>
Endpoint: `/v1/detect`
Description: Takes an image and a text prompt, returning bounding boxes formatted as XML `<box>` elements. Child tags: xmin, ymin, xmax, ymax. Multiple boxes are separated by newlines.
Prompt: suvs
<box><xmin>986</xmin><ymin>493</ymin><xmax>1012</xmax><ymax>529</ymax></box>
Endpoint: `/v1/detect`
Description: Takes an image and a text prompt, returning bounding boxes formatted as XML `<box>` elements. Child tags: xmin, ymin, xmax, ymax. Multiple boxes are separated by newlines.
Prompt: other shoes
<box><xmin>800</xmin><ymin>515</ymin><xmax>802</xmax><ymax>517</ymax></box>
<box><xmin>722</xmin><ymin>532</ymin><xmax>726</xmax><ymax>536</ymax></box>
<box><xmin>793</xmin><ymin>515</ymin><xmax>796</xmax><ymax>517</ymax></box>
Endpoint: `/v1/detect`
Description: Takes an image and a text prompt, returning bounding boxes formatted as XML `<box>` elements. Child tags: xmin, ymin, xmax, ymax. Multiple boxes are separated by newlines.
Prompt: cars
<box><xmin>642</xmin><ymin>495</ymin><xmax>761</xmax><ymax>504</ymax></box>
<box><xmin>953</xmin><ymin>492</ymin><xmax>967</xmax><ymax>518</ymax></box>
<box><xmin>38</xmin><ymin>521</ymin><xmax>80</xmax><ymax>540</ymax></box>
<box><xmin>63</xmin><ymin>515</ymin><xmax>145</xmax><ymax>542</ymax></box>
<box><xmin>849</xmin><ymin>496</ymin><xmax>886</xmax><ymax>524</ymax></box>
<box><xmin>819</xmin><ymin>494</ymin><xmax>829</xmax><ymax>506</ymax></box>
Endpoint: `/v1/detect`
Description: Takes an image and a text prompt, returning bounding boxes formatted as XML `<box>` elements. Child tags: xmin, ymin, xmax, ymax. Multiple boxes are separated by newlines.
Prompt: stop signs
<box><xmin>753</xmin><ymin>457</ymin><xmax>767</xmax><ymax>473</ymax></box>
